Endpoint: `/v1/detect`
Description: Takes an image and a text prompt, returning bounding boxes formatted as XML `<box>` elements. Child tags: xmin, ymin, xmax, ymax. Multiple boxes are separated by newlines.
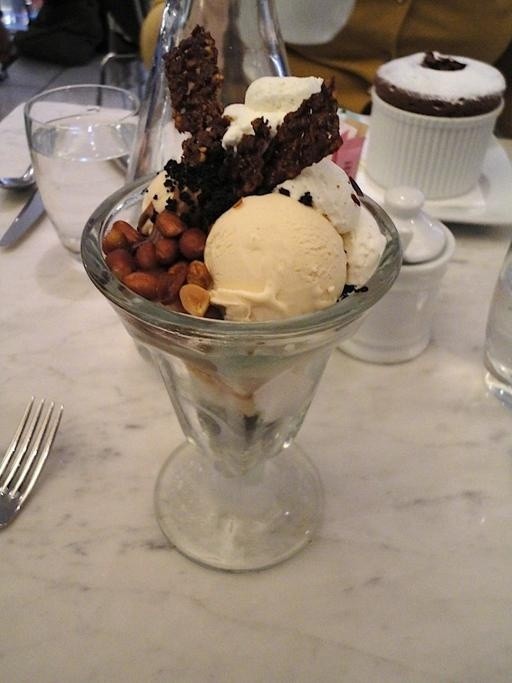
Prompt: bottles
<box><xmin>339</xmin><ymin>221</ymin><xmax>457</xmax><ymax>366</ymax></box>
<box><xmin>129</xmin><ymin>0</ymin><xmax>290</xmax><ymax>179</ymax></box>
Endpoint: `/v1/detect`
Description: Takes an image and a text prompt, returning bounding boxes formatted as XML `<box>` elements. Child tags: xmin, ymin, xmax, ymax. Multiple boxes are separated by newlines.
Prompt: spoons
<box><xmin>0</xmin><ymin>164</ymin><xmax>33</xmax><ymax>193</ymax></box>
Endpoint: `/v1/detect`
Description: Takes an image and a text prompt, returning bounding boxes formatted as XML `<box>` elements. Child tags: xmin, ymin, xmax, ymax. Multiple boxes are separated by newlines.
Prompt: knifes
<box><xmin>0</xmin><ymin>188</ymin><xmax>45</xmax><ymax>248</ymax></box>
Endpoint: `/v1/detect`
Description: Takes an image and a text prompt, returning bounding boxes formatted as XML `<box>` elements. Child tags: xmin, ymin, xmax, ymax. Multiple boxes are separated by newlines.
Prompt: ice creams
<box><xmin>104</xmin><ymin>24</ymin><xmax>388</xmax><ymax>328</ymax></box>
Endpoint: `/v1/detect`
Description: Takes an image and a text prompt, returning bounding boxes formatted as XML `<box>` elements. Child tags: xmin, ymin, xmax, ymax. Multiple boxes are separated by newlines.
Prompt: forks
<box><xmin>0</xmin><ymin>395</ymin><xmax>64</xmax><ymax>531</ymax></box>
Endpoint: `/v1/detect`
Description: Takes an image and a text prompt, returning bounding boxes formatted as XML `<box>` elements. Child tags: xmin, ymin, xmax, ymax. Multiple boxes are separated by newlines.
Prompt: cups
<box><xmin>481</xmin><ymin>245</ymin><xmax>511</xmax><ymax>412</ymax></box>
<box><xmin>22</xmin><ymin>84</ymin><xmax>141</xmax><ymax>254</ymax></box>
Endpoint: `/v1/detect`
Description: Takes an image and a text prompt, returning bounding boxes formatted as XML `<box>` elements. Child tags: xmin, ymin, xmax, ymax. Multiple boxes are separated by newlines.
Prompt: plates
<box><xmin>354</xmin><ymin>126</ymin><xmax>512</xmax><ymax>226</ymax></box>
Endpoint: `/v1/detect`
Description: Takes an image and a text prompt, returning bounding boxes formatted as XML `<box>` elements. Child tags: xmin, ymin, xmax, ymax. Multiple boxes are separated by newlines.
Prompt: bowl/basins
<box><xmin>363</xmin><ymin>88</ymin><xmax>504</xmax><ymax>198</ymax></box>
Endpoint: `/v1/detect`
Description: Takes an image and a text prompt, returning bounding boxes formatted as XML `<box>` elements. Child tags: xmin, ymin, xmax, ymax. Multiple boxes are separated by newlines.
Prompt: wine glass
<box><xmin>81</xmin><ymin>165</ymin><xmax>405</xmax><ymax>576</ymax></box>
